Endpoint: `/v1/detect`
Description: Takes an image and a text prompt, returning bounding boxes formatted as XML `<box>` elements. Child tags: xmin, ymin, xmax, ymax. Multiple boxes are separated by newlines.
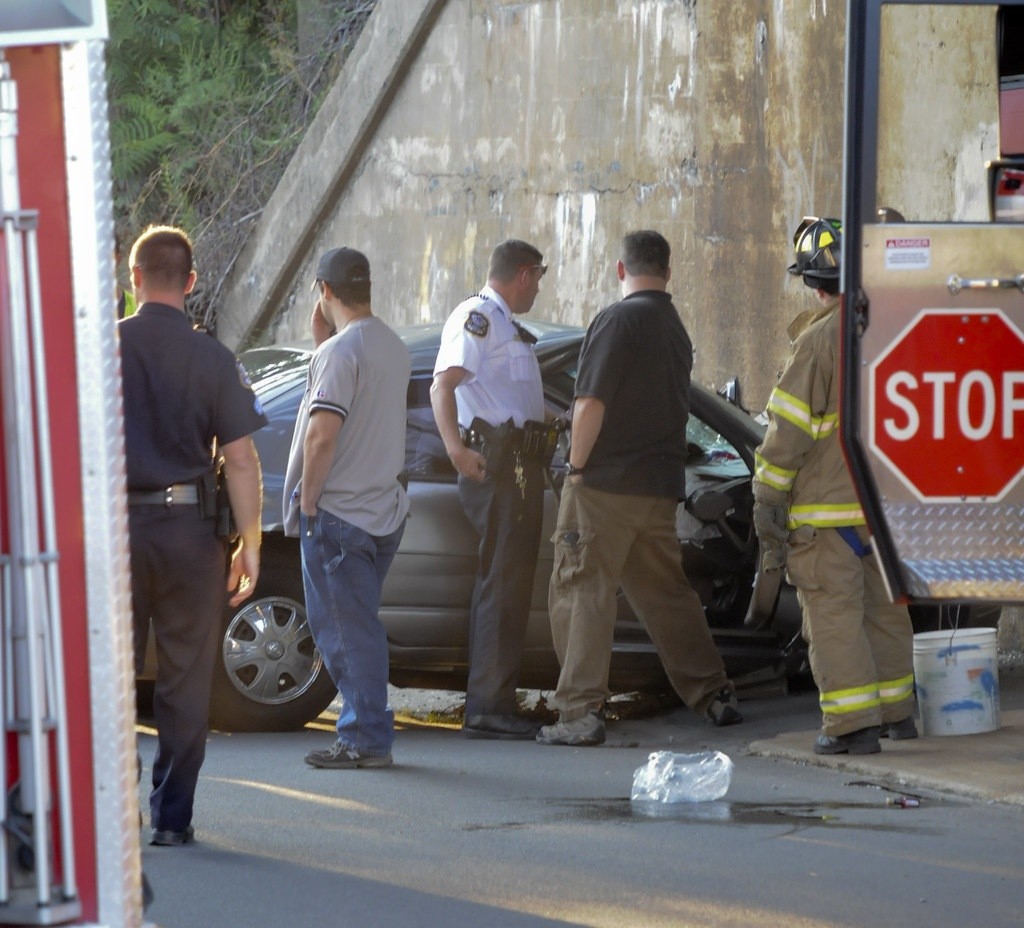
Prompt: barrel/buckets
<box><xmin>913</xmin><ymin>625</ymin><xmax>1001</xmax><ymax>737</ymax></box>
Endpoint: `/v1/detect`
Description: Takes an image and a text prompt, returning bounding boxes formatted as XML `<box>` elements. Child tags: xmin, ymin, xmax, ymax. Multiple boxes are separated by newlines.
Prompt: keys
<box><xmin>515</xmin><ymin>453</ymin><xmax>527</xmax><ymax>499</ymax></box>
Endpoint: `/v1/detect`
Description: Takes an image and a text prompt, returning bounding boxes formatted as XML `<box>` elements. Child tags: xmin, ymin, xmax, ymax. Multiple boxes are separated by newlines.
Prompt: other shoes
<box><xmin>151</xmin><ymin>822</ymin><xmax>194</xmax><ymax>844</ymax></box>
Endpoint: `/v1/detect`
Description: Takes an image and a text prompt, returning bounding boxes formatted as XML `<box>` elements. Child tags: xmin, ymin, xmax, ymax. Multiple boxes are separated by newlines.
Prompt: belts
<box><xmin>128</xmin><ymin>484</ymin><xmax>199</xmax><ymax>505</ymax></box>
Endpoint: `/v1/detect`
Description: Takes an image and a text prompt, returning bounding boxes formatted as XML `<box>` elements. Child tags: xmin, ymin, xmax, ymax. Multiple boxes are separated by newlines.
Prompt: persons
<box><xmin>117</xmin><ymin>225</ymin><xmax>271</xmax><ymax>848</ymax></box>
<box><xmin>283</xmin><ymin>215</ymin><xmax>920</xmax><ymax>770</ymax></box>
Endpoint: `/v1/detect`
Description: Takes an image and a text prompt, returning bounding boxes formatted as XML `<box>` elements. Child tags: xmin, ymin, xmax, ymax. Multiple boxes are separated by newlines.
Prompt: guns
<box><xmin>468</xmin><ymin>417</ymin><xmax>511</xmax><ymax>476</ymax></box>
<box><xmin>215</xmin><ymin>463</ymin><xmax>239</xmax><ymax>544</ymax></box>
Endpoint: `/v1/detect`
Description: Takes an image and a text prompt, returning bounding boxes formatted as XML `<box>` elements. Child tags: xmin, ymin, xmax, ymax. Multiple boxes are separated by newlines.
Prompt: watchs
<box><xmin>563</xmin><ymin>462</ymin><xmax>587</xmax><ymax>475</ymax></box>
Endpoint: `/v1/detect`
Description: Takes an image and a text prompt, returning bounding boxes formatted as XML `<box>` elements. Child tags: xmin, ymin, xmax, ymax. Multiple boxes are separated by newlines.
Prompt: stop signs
<box><xmin>869</xmin><ymin>308</ymin><xmax>1024</xmax><ymax>504</ymax></box>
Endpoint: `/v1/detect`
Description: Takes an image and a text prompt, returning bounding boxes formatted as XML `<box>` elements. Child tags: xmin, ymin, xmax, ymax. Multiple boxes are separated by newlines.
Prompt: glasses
<box><xmin>520</xmin><ymin>265</ymin><xmax>547</xmax><ymax>275</ymax></box>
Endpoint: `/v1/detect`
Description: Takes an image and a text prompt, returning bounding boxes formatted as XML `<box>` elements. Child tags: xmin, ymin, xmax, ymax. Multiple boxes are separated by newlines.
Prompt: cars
<box><xmin>135</xmin><ymin>317</ymin><xmax>804</xmax><ymax>733</ymax></box>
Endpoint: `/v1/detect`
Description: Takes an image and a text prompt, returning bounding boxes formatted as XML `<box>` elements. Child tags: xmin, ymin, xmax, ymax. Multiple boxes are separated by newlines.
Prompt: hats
<box><xmin>310</xmin><ymin>246</ymin><xmax>370</xmax><ymax>293</ymax></box>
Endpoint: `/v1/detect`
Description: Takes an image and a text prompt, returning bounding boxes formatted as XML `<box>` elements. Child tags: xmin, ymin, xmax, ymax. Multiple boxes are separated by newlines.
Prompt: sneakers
<box><xmin>814</xmin><ymin>726</ymin><xmax>881</xmax><ymax>754</ymax></box>
<box><xmin>880</xmin><ymin>717</ymin><xmax>917</xmax><ymax>739</ymax></box>
<box><xmin>465</xmin><ymin>713</ymin><xmax>543</xmax><ymax>740</ymax></box>
<box><xmin>536</xmin><ymin>710</ymin><xmax>606</xmax><ymax>745</ymax></box>
<box><xmin>704</xmin><ymin>688</ymin><xmax>743</xmax><ymax>726</ymax></box>
<box><xmin>304</xmin><ymin>741</ymin><xmax>392</xmax><ymax>768</ymax></box>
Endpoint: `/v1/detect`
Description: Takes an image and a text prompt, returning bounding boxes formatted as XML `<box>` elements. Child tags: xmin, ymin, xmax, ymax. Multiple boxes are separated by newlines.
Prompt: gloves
<box><xmin>753</xmin><ymin>501</ymin><xmax>790</xmax><ymax>573</ymax></box>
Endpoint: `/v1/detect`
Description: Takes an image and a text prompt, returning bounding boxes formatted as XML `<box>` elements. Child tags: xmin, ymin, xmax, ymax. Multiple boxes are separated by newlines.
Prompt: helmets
<box><xmin>787</xmin><ymin>216</ymin><xmax>841</xmax><ymax>278</ymax></box>
